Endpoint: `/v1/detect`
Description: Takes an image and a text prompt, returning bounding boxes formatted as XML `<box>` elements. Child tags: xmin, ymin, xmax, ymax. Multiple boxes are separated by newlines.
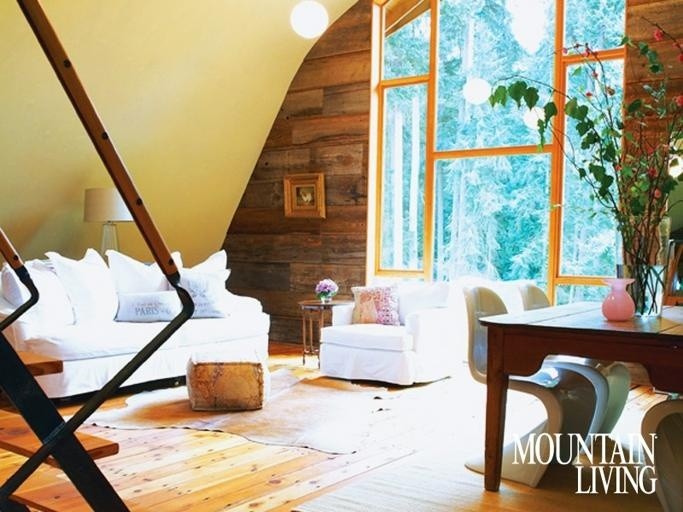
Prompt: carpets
<box><xmin>80</xmin><ymin>368</ymin><xmax>395</xmax><ymax>456</ymax></box>
<box><xmin>291</xmin><ymin>445</ymin><xmax>663</xmax><ymax>511</ymax></box>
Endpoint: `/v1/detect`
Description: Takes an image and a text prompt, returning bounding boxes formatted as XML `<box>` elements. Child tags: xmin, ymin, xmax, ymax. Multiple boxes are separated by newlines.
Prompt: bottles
<box><xmin>601</xmin><ymin>276</ymin><xmax>635</xmax><ymax>323</ymax></box>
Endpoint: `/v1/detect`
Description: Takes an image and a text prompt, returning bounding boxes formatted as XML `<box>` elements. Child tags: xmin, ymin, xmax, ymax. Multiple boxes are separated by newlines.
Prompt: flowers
<box><xmin>488</xmin><ymin>11</ymin><xmax>683</xmax><ymax>313</ymax></box>
<box><xmin>314</xmin><ymin>278</ymin><xmax>339</xmax><ymax>298</ymax></box>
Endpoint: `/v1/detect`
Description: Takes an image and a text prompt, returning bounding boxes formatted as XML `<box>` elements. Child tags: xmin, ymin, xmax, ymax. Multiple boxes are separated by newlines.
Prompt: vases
<box><xmin>617</xmin><ymin>264</ymin><xmax>668</xmax><ymax>316</ymax></box>
<box><xmin>320</xmin><ymin>296</ymin><xmax>332</xmax><ymax>303</ymax></box>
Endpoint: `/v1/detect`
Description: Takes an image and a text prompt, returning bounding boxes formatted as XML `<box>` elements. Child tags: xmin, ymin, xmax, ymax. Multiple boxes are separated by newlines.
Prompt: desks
<box><xmin>477</xmin><ymin>301</ymin><xmax>683</xmax><ymax>492</ymax></box>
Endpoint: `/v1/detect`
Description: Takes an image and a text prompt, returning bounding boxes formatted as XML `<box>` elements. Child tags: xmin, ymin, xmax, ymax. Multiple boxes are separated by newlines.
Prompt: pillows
<box><xmin>0</xmin><ymin>247</ymin><xmax>233</xmax><ymax>350</ymax></box>
<box><xmin>349</xmin><ymin>283</ymin><xmax>400</xmax><ymax>325</ymax></box>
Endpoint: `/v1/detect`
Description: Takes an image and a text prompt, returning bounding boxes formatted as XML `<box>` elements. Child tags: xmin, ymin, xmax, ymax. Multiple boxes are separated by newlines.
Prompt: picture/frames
<box><xmin>284</xmin><ymin>172</ymin><xmax>326</xmax><ymax>219</ymax></box>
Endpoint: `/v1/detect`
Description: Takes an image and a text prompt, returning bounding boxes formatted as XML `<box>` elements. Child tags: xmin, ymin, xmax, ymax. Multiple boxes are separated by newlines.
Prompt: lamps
<box><xmin>84</xmin><ymin>188</ymin><xmax>134</xmax><ymax>263</ymax></box>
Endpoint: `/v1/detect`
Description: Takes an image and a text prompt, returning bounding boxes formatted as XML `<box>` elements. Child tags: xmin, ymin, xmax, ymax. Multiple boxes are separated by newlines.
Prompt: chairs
<box><xmin>320</xmin><ymin>280</ymin><xmax>463</xmax><ymax>386</ymax></box>
<box><xmin>464</xmin><ymin>281</ymin><xmax>632</xmax><ymax>488</ymax></box>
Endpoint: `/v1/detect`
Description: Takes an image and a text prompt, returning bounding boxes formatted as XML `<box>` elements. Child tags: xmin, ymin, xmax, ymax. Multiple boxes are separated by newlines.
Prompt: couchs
<box><xmin>1</xmin><ymin>272</ymin><xmax>270</xmax><ymax>402</ymax></box>
